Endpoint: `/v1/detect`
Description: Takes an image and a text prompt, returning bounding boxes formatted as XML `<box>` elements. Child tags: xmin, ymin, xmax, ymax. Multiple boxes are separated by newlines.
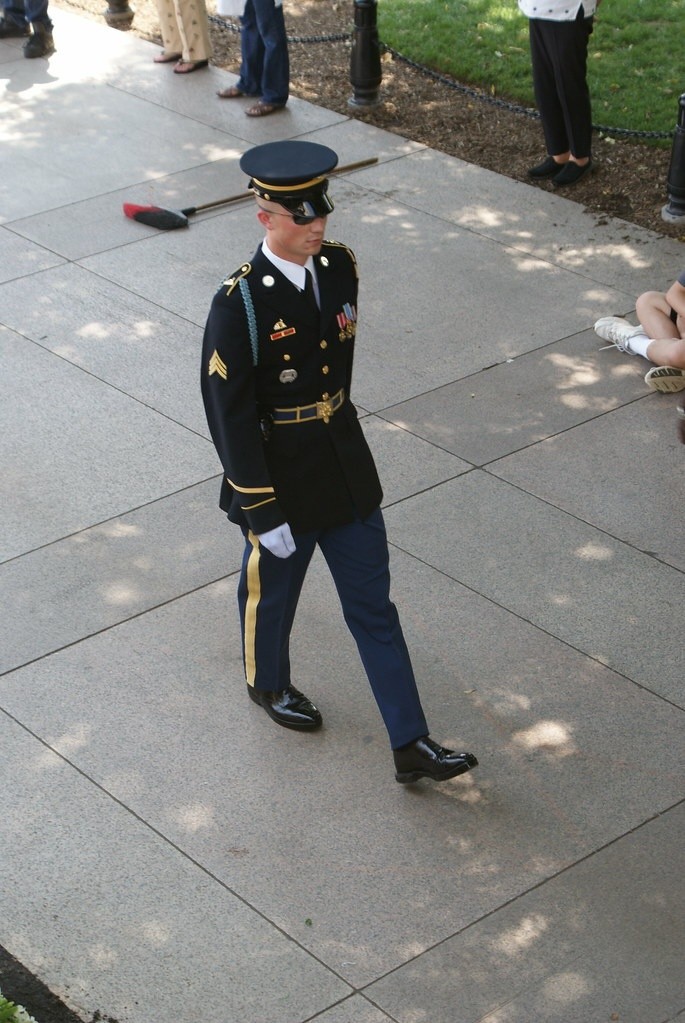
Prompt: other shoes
<box><xmin>552</xmin><ymin>158</ymin><xmax>592</xmax><ymax>187</ymax></box>
<box><xmin>25</xmin><ymin>21</ymin><xmax>54</xmax><ymax>58</ymax></box>
<box><xmin>0</xmin><ymin>17</ymin><xmax>30</xmax><ymax>39</ymax></box>
<box><xmin>528</xmin><ymin>155</ymin><xmax>569</xmax><ymax>180</ymax></box>
<box><xmin>153</xmin><ymin>52</ymin><xmax>181</xmax><ymax>63</ymax></box>
<box><xmin>173</xmin><ymin>58</ymin><xmax>209</xmax><ymax>74</ymax></box>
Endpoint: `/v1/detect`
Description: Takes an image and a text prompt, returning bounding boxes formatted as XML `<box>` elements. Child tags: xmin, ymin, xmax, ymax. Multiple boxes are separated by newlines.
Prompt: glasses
<box><xmin>258</xmin><ymin>206</ymin><xmax>328</xmax><ymax>224</ymax></box>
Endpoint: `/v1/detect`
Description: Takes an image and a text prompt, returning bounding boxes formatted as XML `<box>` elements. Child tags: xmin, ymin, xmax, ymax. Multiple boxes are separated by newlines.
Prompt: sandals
<box><xmin>216</xmin><ymin>86</ymin><xmax>251</xmax><ymax>98</ymax></box>
<box><xmin>245</xmin><ymin>100</ymin><xmax>284</xmax><ymax>116</ymax></box>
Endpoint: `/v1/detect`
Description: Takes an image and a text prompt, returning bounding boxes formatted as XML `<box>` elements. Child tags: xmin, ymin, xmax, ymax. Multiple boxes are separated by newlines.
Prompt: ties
<box><xmin>298</xmin><ymin>268</ymin><xmax>320</xmax><ymax>312</ymax></box>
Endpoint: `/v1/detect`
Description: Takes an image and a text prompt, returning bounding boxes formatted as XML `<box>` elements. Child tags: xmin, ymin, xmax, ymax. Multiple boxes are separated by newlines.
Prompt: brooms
<box><xmin>122</xmin><ymin>157</ymin><xmax>379</xmax><ymax>232</ymax></box>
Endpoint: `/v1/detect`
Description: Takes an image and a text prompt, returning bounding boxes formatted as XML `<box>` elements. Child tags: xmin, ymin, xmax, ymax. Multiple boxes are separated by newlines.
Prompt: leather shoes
<box><xmin>247</xmin><ymin>683</ymin><xmax>322</xmax><ymax>730</ymax></box>
<box><xmin>394</xmin><ymin>736</ymin><xmax>479</xmax><ymax>783</ymax></box>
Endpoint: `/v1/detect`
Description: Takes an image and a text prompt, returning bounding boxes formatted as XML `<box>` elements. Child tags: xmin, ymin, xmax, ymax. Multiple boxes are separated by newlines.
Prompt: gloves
<box><xmin>258</xmin><ymin>522</ymin><xmax>296</xmax><ymax>559</ymax></box>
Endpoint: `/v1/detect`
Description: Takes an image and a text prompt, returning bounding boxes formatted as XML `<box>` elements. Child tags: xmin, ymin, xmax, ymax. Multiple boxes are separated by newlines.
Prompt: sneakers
<box><xmin>593</xmin><ymin>317</ymin><xmax>647</xmax><ymax>357</ymax></box>
<box><xmin>644</xmin><ymin>365</ymin><xmax>685</xmax><ymax>393</ymax></box>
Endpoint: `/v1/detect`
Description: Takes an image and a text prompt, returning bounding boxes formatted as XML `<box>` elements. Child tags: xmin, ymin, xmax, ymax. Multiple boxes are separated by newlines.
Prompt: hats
<box><xmin>239</xmin><ymin>141</ymin><xmax>338</xmax><ymax>218</ymax></box>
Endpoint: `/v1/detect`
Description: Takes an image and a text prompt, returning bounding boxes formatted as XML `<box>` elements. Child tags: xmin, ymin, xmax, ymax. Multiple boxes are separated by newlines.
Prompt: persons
<box><xmin>593</xmin><ymin>270</ymin><xmax>685</xmax><ymax>393</ymax></box>
<box><xmin>517</xmin><ymin>0</ymin><xmax>602</xmax><ymax>188</ymax></box>
<box><xmin>0</xmin><ymin>0</ymin><xmax>55</xmax><ymax>59</ymax></box>
<box><xmin>151</xmin><ymin>0</ymin><xmax>214</xmax><ymax>73</ymax></box>
<box><xmin>216</xmin><ymin>0</ymin><xmax>290</xmax><ymax>116</ymax></box>
<box><xmin>200</xmin><ymin>141</ymin><xmax>478</xmax><ymax>784</ymax></box>
